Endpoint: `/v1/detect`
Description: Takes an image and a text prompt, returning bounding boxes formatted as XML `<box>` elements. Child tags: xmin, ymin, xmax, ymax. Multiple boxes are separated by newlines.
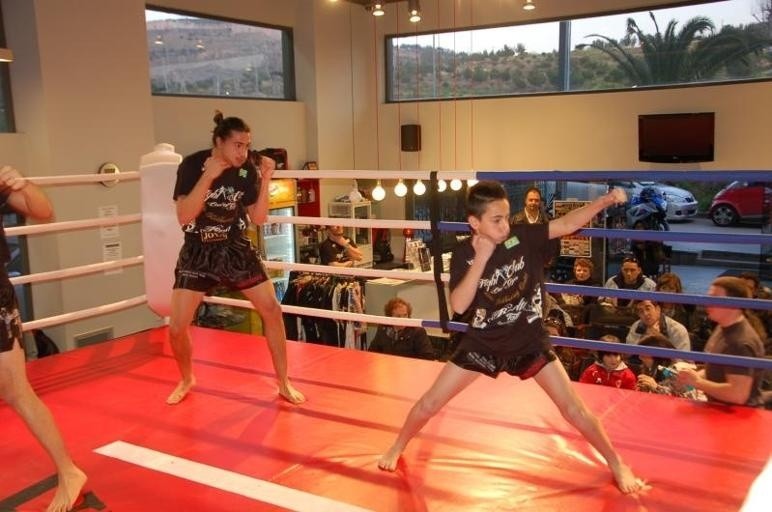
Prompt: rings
<box><xmin>13</xmin><ymin>177</ymin><xmax>17</xmax><ymax>182</ymax></box>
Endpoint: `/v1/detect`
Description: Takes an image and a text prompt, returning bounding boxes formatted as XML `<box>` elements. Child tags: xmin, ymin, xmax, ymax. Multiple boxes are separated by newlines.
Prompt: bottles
<box><xmin>297</xmin><ymin>182</ymin><xmax>316</xmax><ymax>203</ymax></box>
<box><xmin>262</xmin><ymin>223</ymin><xmax>285</xmax><ymax>301</ymax></box>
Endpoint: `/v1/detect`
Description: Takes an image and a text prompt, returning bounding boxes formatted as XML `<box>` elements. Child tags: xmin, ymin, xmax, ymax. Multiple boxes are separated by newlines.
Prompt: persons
<box><xmin>163</xmin><ymin>110</ymin><xmax>309</xmax><ymax>406</ymax></box>
<box><xmin>510</xmin><ymin>188</ymin><xmax>560</xmax><ymax>269</ymax></box>
<box><xmin>367</xmin><ymin>296</ymin><xmax>434</xmax><ymax>358</ymax></box>
<box><xmin>1</xmin><ymin>162</ymin><xmax>92</xmax><ymax>509</ymax></box>
<box><xmin>533</xmin><ymin>259</ymin><xmax>772</xmax><ymax>409</ymax></box>
<box><xmin>375</xmin><ymin>180</ymin><xmax>646</xmax><ymax>496</ymax></box>
<box><xmin>318</xmin><ymin>223</ymin><xmax>362</xmax><ymax>267</ymax></box>
<box><xmin>640</xmin><ymin>186</ymin><xmax>670</xmax><ymax>237</ymax></box>
<box><xmin>633</xmin><ymin>221</ymin><xmax>660</xmax><ymax>276</ymax></box>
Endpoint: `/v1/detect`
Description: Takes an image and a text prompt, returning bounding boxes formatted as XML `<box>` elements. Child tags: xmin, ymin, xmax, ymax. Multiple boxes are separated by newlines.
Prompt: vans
<box><xmin>708</xmin><ymin>181</ymin><xmax>772</xmax><ymax>227</ymax></box>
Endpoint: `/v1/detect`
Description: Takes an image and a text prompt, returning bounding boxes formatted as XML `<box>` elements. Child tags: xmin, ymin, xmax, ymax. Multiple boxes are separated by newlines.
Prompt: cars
<box><xmin>624</xmin><ymin>182</ymin><xmax>699</xmax><ymax>221</ymax></box>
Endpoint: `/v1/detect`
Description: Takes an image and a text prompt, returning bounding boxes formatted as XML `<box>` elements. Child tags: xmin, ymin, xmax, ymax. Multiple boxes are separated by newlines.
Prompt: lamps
<box><xmin>347</xmin><ymin>1</ymin><xmax>479</xmax><ymax>205</ymax></box>
